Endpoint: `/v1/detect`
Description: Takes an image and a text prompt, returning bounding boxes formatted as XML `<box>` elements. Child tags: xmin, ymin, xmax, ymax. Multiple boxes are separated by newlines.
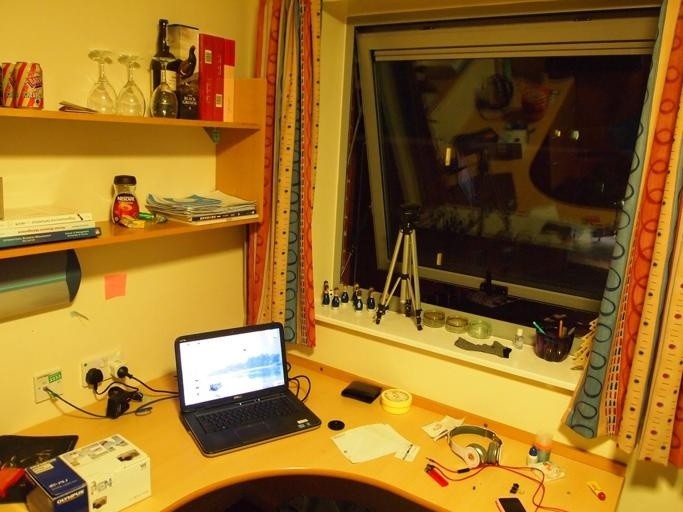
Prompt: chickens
<box><xmin>176</xmin><ymin>45</ymin><xmax>197</xmax><ymax>87</ymax></box>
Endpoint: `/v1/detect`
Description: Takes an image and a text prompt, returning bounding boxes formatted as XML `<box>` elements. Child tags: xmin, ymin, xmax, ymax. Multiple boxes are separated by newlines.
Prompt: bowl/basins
<box><xmin>380</xmin><ymin>388</ymin><xmax>412</xmax><ymax>415</ymax></box>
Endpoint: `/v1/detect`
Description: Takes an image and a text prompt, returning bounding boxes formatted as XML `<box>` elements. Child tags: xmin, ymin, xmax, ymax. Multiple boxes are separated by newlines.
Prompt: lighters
<box><xmin>425</xmin><ymin>467</ymin><xmax>449</xmax><ymax>487</ymax></box>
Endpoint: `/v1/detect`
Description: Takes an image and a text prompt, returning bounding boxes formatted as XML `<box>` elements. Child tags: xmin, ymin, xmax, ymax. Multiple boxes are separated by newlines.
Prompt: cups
<box><xmin>533</xmin><ymin>323</ymin><xmax>573</xmax><ymax>363</ymax></box>
<box><xmin>468</xmin><ymin>318</ymin><xmax>492</xmax><ymax>339</ymax></box>
<box><xmin>445</xmin><ymin>315</ymin><xmax>467</xmax><ymax>334</ymax></box>
<box><xmin>422</xmin><ymin>308</ymin><xmax>445</xmax><ymax>328</ymax></box>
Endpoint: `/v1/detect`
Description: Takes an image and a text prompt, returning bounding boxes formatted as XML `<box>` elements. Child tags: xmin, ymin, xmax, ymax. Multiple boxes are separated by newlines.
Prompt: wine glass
<box><xmin>149</xmin><ymin>56</ymin><xmax>177</xmax><ymax>118</ymax></box>
<box><xmin>116</xmin><ymin>55</ymin><xmax>146</xmax><ymax>116</ymax></box>
<box><xmin>86</xmin><ymin>51</ymin><xmax>118</xmax><ymax>115</ymax></box>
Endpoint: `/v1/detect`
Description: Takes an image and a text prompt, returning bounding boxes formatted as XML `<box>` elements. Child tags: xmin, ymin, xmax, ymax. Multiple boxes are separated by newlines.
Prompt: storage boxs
<box><xmin>21</xmin><ymin>432</ymin><xmax>153</xmax><ymax>512</ymax></box>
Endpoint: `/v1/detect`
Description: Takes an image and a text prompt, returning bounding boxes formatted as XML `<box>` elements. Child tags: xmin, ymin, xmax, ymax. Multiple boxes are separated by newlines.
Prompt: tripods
<box><xmin>376</xmin><ymin>204</ymin><xmax>423</xmax><ymax>330</ymax></box>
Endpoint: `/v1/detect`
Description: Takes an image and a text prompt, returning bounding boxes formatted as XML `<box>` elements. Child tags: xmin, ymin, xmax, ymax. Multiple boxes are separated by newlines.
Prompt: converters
<box><xmin>108</xmin><ymin>390</ymin><xmax>129</xmax><ymax>419</ymax></box>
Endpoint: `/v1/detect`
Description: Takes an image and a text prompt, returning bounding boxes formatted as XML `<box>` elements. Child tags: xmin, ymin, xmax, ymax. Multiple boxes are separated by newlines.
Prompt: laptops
<box><xmin>174</xmin><ymin>322</ymin><xmax>322</xmax><ymax>458</ymax></box>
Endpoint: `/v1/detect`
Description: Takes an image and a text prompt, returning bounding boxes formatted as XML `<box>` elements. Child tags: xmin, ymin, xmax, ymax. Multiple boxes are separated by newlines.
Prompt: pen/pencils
<box><xmin>533</xmin><ymin>319</ymin><xmax>576</xmax><ymax>338</ymax></box>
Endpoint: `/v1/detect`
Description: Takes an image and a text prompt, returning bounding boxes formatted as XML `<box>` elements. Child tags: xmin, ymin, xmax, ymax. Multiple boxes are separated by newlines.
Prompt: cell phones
<box><xmin>496</xmin><ymin>496</ymin><xmax>529</xmax><ymax>512</ymax></box>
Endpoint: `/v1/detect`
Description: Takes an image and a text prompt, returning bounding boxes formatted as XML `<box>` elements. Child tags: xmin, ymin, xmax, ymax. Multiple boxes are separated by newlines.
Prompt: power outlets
<box><xmin>77</xmin><ymin>349</ymin><xmax>126</xmax><ymax>391</ymax></box>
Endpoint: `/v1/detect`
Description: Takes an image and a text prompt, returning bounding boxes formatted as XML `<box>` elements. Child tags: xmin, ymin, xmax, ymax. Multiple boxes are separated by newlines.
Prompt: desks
<box><xmin>0</xmin><ymin>347</ymin><xmax>635</xmax><ymax>512</ymax></box>
<box><xmin>427</xmin><ymin>55</ymin><xmax>621</xmax><ymax>262</ymax></box>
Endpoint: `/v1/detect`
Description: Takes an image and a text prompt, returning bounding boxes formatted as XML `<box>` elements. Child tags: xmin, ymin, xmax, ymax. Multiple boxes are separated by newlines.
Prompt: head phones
<box><xmin>448</xmin><ymin>425</ymin><xmax>504</xmax><ymax>468</ymax></box>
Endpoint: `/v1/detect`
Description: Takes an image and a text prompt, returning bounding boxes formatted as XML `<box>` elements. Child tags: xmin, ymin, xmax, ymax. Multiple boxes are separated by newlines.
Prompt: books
<box><xmin>0</xmin><ymin>206</ymin><xmax>101</xmax><ymax>248</ymax></box>
<box><xmin>146</xmin><ymin>189</ymin><xmax>256</xmax><ymax>222</ymax></box>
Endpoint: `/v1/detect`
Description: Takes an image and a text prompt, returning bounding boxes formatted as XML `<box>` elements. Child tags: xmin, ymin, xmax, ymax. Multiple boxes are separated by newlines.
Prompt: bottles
<box><xmin>536</xmin><ymin>437</ymin><xmax>551</xmax><ymax>463</ymax></box>
<box><xmin>526</xmin><ymin>447</ymin><xmax>537</xmax><ymax>466</ymax></box>
<box><xmin>109</xmin><ymin>175</ymin><xmax>139</xmax><ymax>226</ymax></box>
<box><xmin>512</xmin><ymin>328</ymin><xmax>525</xmax><ymax>350</ymax></box>
<box><xmin>150</xmin><ymin>19</ymin><xmax>178</xmax><ymax>119</ymax></box>
<box><xmin>321</xmin><ymin>282</ymin><xmax>375</xmax><ymax>312</ymax></box>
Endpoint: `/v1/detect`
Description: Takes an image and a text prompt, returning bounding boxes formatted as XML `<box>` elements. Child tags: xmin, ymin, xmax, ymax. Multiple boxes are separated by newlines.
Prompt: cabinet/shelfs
<box><xmin>0</xmin><ymin>73</ymin><xmax>271</xmax><ymax>262</ymax></box>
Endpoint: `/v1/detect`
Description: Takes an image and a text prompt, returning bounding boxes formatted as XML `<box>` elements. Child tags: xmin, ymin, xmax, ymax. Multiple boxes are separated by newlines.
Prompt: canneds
<box><xmin>2</xmin><ymin>63</ymin><xmax>16</xmax><ymax>108</ymax></box>
<box><xmin>14</xmin><ymin>62</ymin><xmax>44</xmax><ymax>108</ymax></box>
